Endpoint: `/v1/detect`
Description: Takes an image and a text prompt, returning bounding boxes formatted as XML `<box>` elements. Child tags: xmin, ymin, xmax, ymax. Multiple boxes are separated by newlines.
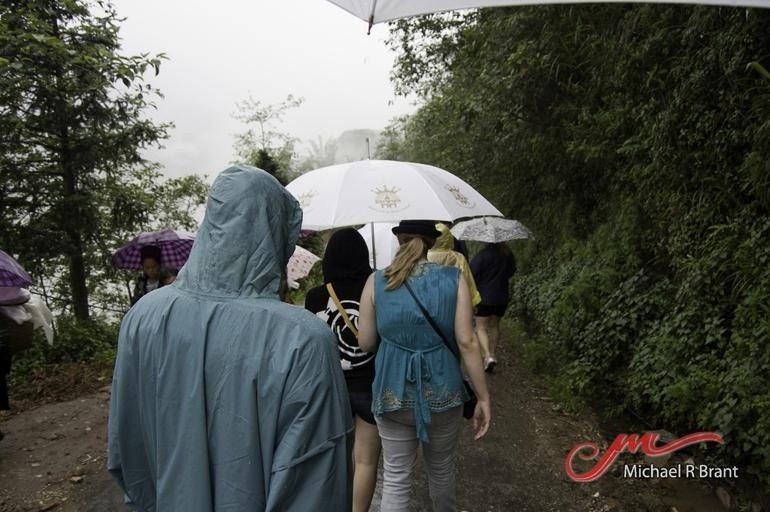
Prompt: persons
<box><xmin>303</xmin><ymin>228</ymin><xmax>380</xmax><ymax>511</ymax></box>
<box><xmin>426</xmin><ymin>222</ymin><xmax>482</xmax><ymax>308</ymax></box>
<box><xmin>131</xmin><ymin>243</ymin><xmax>170</xmax><ymax>298</ymax></box>
<box><xmin>1</xmin><ymin>305</ymin><xmax>35</xmax><ymax>416</ymax></box>
<box><xmin>467</xmin><ymin>243</ymin><xmax>517</xmax><ymax>373</ymax></box>
<box><xmin>358</xmin><ymin>218</ymin><xmax>495</xmax><ymax>512</ymax></box>
<box><xmin>106</xmin><ymin>164</ymin><xmax>353</xmax><ymax>512</ymax></box>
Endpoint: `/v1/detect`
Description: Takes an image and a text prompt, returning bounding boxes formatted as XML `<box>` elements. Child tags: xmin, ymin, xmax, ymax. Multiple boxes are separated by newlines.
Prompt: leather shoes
<box><xmin>484</xmin><ymin>357</ymin><xmax>496</xmax><ymax>372</ymax></box>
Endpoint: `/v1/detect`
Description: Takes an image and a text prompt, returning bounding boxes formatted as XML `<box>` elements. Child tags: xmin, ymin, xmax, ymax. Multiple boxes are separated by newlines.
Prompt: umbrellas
<box><xmin>330</xmin><ymin>0</ymin><xmax>540</xmax><ymax>37</ymax></box>
<box><xmin>287</xmin><ymin>158</ymin><xmax>505</xmax><ymax>269</ymax></box>
<box><xmin>110</xmin><ymin>228</ymin><xmax>196</xmax><ymax>268</ymax></box>
<box><xmin>0</xmin><ymin>250</ymin><xmax>36</xmax><ymax>286</ymax></box>
<box><xmin>450</xmin><ymin>215</ymin><xmax>535</xmax><ymax>242</ymax></box>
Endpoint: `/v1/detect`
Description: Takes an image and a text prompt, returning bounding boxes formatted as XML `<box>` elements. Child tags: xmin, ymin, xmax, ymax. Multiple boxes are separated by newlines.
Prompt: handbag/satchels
<box><xmin>463</xmin><ymin>379</ymin><xmax>477</xmax><ymax>419</ymax></box>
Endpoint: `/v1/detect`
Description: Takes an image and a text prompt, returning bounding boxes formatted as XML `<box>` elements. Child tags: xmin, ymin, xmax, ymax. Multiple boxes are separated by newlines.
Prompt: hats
<box><xmin>392</xmin><ymin>221</ymin><xmax>442</xmax><ymax>238</ymax></box>
